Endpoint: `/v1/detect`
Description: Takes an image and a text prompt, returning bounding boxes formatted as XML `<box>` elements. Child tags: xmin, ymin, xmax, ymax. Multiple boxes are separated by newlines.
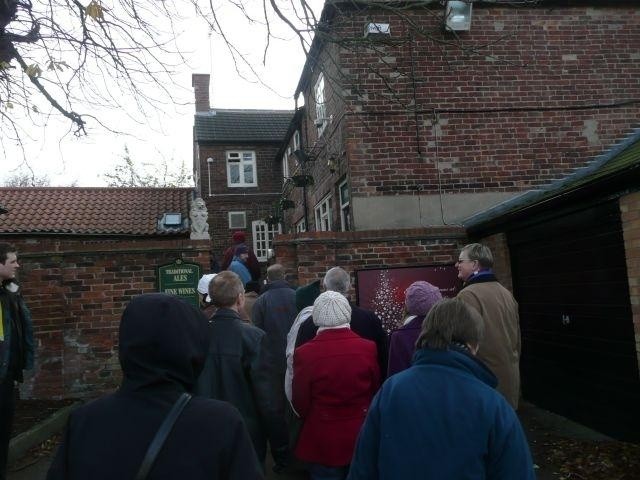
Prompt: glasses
<box><xmin>458</xmin><ymin>258</ymin><xmax>481</xmax><ymax>265</ymax></box>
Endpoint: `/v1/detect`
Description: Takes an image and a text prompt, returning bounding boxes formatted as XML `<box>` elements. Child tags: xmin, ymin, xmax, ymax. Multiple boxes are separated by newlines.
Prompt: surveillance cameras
<box><xmin>314</xmin><ymin>119</ymin><xmax>323</xmax><ymax>129</ymax></box>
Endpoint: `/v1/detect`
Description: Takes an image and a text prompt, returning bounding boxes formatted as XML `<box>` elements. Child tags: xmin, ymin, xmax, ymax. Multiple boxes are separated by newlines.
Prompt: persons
<box><xmin>46</xmin><ymin>293</ymin><xmax>266</xmax><ymax>480</ymax></box>
<box><xmin>295</xmin><ymin>278</ymin><xmax>326</xmax><ymax>314</ymax></box>
<box><xmin>0</xmin><ymin>243</ymin><xmax>35</xmax><ymax>480</ymax></box>
<box><xmin>347</xmin><ymin>297</ymin><xmax>535</xmax><ymax>480</ymax></box>
<box><xmin>252</xmin><ymin>264</ymin><xmax>297</xmax><ymax>369</ymax></box>
<box><xmin>210</xmin><ymin>253</ymin><xmax>220</xmax><ymax>273</ymax></box>
<box><xmin>387</xmin><ymin>280</ymin><xmax>443</xmax><ymax>376</ymax></box>
<box><xmin>227</xmin><ymin>245</ymin><xmax>252</xmax><ymax>293</ymax></box>
<box><xmin>243</xmin><ymin>281</ymin><xmax>261</xmax><ymax>325</ymax></box>
<box><xmin>454</xmin><ymin>243</ymin><xmax>521</xmax><ymax>412</ymax></box>
<box><xmin>190</xmin><ymin>271</ymin><xmax>289</xmax><ymax>476</ymax></box>
<box><xmin>291</xmin><ymin>290</ymin><xmax>381</xmax><ymax>480</ymax></box>
<box><xmin>294</xmin><ymin>266</ymin><xmax>389</xmax><ymax>388</ymax></box>
<box><xmin>221</xmin><ymin>231</ymin><xmax>262</xmax><ymax>280</ymax></box>
<box><xmin>196</xmin><ymin>273</ymin><xmax>252</xmax><ymax>324</ymax></box>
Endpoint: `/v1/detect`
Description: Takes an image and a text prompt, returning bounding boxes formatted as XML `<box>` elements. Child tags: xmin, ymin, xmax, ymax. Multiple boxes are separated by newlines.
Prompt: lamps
<box><xmin>441</xmin><ymin>0</ymin><xmax>473</xmax><ymax>34</ymax></box>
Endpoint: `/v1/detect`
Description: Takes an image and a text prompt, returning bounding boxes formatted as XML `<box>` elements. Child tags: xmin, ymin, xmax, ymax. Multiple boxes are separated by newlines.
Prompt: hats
<box><xmin>313</xmin><ymin>291</ymin><xmax>352</xmax><ymax>327</ymax></box>
<box><xmin>233</xmin><ymin>231</ymin><xmax>246</xmax><ymax>243</ymax></box>
<box><xmin>405</xmin><ymin>281</ymin><xmax>443</xmax><ymax>315</ymax></box>
<box><xmin>197</xmin><ymin>274</ymin><xmax>217</xmax><ymax>303</ymax></box>
<box><xmin>235</xmin><ymin>244</ymin><xmax>248</xmax><ymax>257</ymax></box>
